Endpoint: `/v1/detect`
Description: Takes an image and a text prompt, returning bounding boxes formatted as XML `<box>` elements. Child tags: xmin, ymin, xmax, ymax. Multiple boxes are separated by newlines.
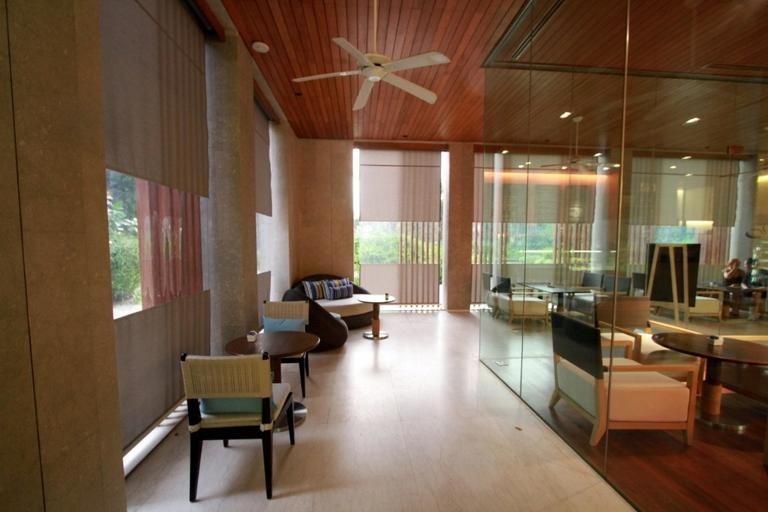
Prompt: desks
<box><xmin>357</xmin><ymin>294</ymin><xmax>396</xmax><ymax>340</ymax></box>
<box><xmin>226</xmin><ymin>331</ymin><xmax>321</xmax><ymax>431</ymax></box>
<box><xmin>651</xmin><ymin>332</ymin><xmax>768</xmax><ymax>431</ymax></box>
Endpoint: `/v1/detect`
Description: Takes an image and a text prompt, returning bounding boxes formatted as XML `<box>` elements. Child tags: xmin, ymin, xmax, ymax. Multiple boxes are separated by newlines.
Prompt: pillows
<box><xmin>262</xmin><ymin>315</ymin><xmax>306</xmax><ymax>333</ymax></box>
<box><xmin>301</xmin><ymin>276</ymin><xmax>353</xmax><ymax>300</ymax></box>
<box><xmin>200</xmin><ymin>371</ymin><xmax>278</xmax><ymax>414</ymax></box>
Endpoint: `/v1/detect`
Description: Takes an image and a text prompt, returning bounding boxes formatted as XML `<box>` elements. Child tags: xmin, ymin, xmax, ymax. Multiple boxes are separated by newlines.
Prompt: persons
<box><xmin>729</xmin><ymin>257</ymin><xmax>768</xmax><ymax>321</ymax></box>
<box><xmin>721</xmin><ymin>258</ymin><xmax>744</xmax><ymax>285</ymax></box>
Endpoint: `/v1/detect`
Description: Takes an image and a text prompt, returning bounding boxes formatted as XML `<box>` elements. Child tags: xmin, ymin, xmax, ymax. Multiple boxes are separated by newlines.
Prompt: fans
<box><xmin>541</xmin><ymin>115</ymin><xmax>619</xmax><ymax>168</ymax></box>
<box><xmin>292</xmin><ymin>0</ymin><xmax>452</xmax><ymax>112</ymax></box>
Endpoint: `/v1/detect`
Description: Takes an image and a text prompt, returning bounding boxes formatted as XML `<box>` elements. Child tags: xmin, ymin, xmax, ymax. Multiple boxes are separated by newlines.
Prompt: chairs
<box><xmin>180</xmin><ymin>351</ymin><xmax>294</xmax><ymax>502</ymax></box>
<box><xmin>282</xmin><ymin>289</ymin><xmax>348</xmax><ymax>353</ymax></box>
<box><xmin>262</xmin><ymin>299</ymin><xmax>310</xmax><ymax>398</ymax></box>
<box><xmin>292</xmin><ymin>273</ymin><xmax>372</xmax><ymax>330</ymax></box>
<box><xmin>549</xmin><ymin>311</ymin><xmax>701</xmax><ymax>446</ymax></box>
<box><xmin>482</xmin><ymin>268</ymin><xmax>768</xmax><ymax>326</ymax></box>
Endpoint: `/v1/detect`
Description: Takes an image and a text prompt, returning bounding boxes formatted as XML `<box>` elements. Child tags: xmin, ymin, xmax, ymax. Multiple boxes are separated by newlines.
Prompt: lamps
<box><xmin>363</xmin><ymin>67</ymin><xmax>386</xmax><ymax>82</ymax></box>
